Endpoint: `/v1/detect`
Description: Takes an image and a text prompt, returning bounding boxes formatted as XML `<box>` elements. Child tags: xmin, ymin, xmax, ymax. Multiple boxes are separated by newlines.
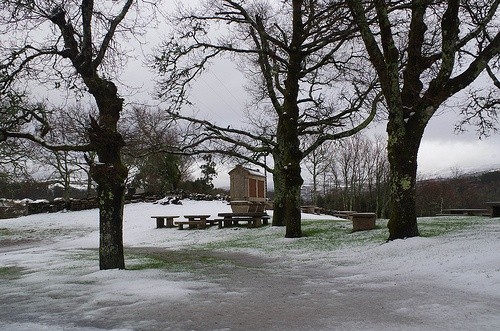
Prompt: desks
<box><xmin>184</xmin><ymin>214</ymin><xmax>211</xmax><ymax>229</ymax></box>
<box><xmin>218</xmin><ymin>211</ymin><xmax>269</xmax><ymax>227</ymax></box>
<box><xmin>150</xmin><ymin>215</ymin><xmax>180</xmax><ymax>228</ymax></box>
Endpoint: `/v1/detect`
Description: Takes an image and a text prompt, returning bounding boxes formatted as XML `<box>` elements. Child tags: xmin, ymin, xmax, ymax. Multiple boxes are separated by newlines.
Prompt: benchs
<box><xmin>232</xmin><ymin>217</ymin><xmax>270</xmax><ymax>225</ymax></box>
<box><xmin>194</xmin><ymin>219</ymin><xmax>218</xmax><ymax>226</ymax></box>
<box><xmin>174</xmin><ymin>220</ymin><xmax>208</xmax><ymax>230</ymax></box>
<box><xmin>214</xmin><ymin>217</ymin><xmax>258</xmax><ymax>229</ymax></box>
